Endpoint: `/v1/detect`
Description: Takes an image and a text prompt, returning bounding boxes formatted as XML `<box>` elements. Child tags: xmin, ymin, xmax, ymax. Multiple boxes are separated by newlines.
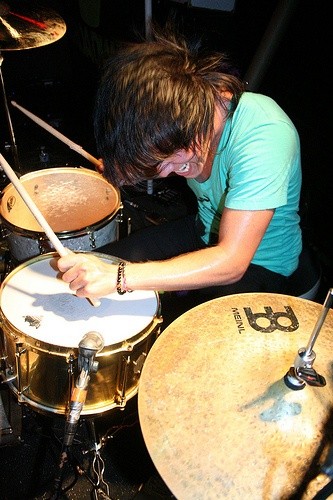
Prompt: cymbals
<box><xmin>0</xmin><ymin>0</ymin><xmax>68</xmax><ymax>52</ymax></box>
<box><xmin>137</xmin><ymin>293</ymin><xmax>333</xmax><ymax>499</ymax></box>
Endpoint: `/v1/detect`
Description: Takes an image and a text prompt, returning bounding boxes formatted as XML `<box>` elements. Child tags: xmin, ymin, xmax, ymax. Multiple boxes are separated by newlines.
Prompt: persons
<box><xmin>55</xmin><ymin>39</ymin><xmax>302</xmax><ymax>332</ymax></box>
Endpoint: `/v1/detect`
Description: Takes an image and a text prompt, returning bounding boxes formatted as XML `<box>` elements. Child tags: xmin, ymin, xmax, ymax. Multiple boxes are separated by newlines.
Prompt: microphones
<box><xmin>65</xmin><ymin>332</ymin><xmax>102</xmax><ymax>447</ymax></box>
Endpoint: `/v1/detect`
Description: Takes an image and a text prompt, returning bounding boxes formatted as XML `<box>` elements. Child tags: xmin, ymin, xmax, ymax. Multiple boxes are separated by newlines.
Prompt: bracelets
<box><xmin>116</xmin><ymin>260</ymin><xmax>133</xmax><ymax>294</ymax></box>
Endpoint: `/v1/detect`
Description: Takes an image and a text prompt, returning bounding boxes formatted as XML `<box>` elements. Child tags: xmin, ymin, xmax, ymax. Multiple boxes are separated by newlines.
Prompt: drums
<box><xmin>0</xmin><ymin>167</ymin><xmax>132</xmax><ymax>266</ymax></box>
<box><xmin>0</xmin><ymin>249</ymin><xmax>164</xmax><ymax>420</ymax></box>
